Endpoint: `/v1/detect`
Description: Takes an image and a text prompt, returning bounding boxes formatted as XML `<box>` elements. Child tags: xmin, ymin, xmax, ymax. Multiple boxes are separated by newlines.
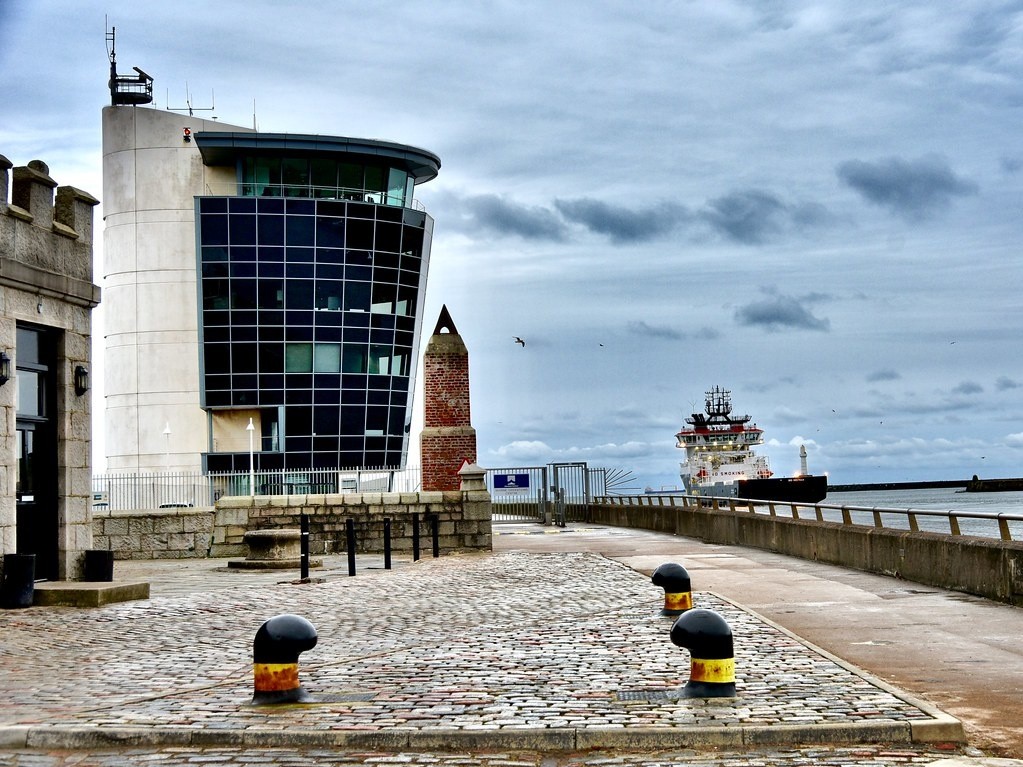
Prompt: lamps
<box><xmin>75</xmin><ymin>366</ymin><xmax>89</xmax><ymax>396</ymax></box>
<box><xmin>0</xmin><ymin>352</ymin><xmax>11</xmax><ymax>386</ymax></box>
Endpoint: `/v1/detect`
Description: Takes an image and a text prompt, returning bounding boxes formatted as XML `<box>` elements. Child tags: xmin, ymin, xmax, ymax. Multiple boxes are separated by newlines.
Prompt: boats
<box><xmin>673</xmin><ymin>384</ymin><xmax>828</xmax><ymax>507</ymax></box>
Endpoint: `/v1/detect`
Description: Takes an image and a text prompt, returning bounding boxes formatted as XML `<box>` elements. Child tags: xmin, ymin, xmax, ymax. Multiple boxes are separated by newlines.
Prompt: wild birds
<box><xmin>816</xmin><ymin>341</ymin><xmax>987</xmax><ymax>462</ymax></box>
<box><xmin>513</xmin><ymin>335</ymin><xmax>526</xmax><ymax>348</ymax></box>
<box><xmin>599</xmin><ymin>343</ymin><xmax>603</xmax><ymax>347</ymax></box>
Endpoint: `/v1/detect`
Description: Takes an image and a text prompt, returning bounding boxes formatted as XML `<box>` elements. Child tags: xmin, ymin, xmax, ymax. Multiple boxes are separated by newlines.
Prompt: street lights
<box><xmin>163</xmin><ymin>421</ymin><xmax>171</xmax><ymax>472</ymax></box>
<box><xmin>245</xmin><ymin>417</ymin><xmax>256</xmax><ymax>497</ymax></box>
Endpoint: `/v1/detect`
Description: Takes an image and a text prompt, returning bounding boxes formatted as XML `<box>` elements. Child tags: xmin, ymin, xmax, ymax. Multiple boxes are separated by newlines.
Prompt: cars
<box><xmin>158</xmin><ymin>502</ymin><xmax>194</xmax><ymax>508</ymax></box>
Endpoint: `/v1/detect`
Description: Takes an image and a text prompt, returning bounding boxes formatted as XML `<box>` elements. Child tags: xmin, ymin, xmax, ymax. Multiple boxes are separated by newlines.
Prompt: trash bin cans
<box><xmin>85</xmin><ymin>549</ymin><xmax>114</xmax><ymax>582</ymax></box>
<box><xmin>2</xmin><ymin>553</ymin><xmax>35</xmax><ymax>608</ymax></box>
